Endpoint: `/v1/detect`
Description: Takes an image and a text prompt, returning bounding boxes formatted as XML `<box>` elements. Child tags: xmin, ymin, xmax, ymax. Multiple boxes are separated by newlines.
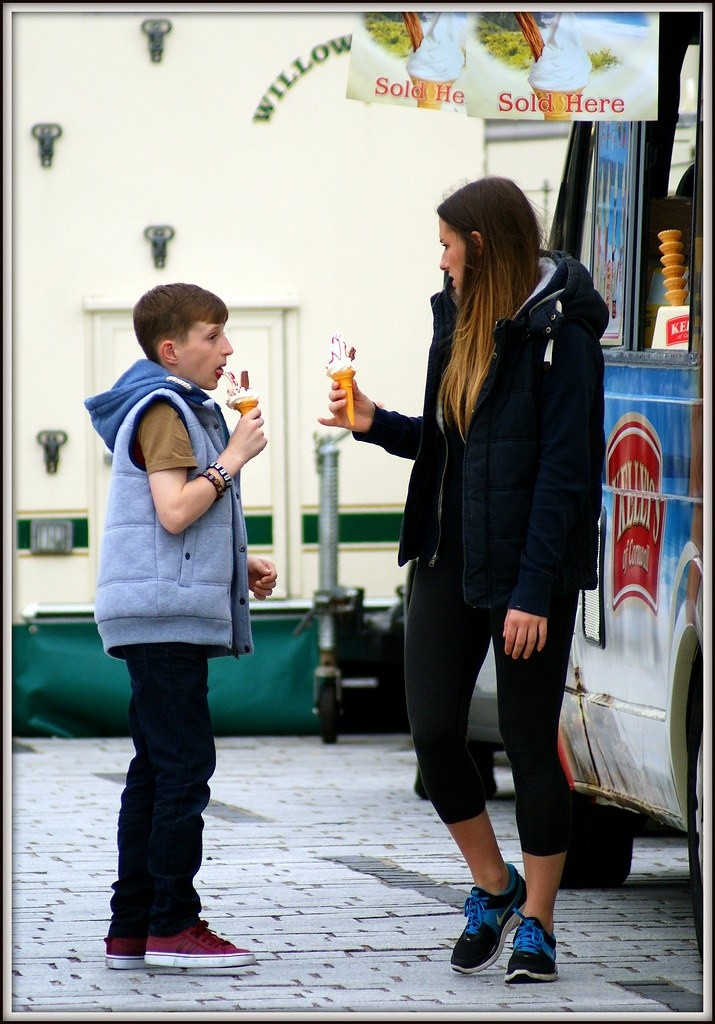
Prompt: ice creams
<box><xmin>326</xmin><ymin>335</ymin><xmax>356</xmax><ymax>426</ymax></box>
<box><xmin>515</xmin><ymin>12</ymin><xmax>588</xmax><ymax>121</ymax></box>
<box><xmin>403</xmin><ymin>12</ymin><xmax>463</xmax><ymax>108</ymax></box>
<box><xmin>223</xmin><ymin>371</ymin><xmax>260</xmax><ymax>427</ymax></box>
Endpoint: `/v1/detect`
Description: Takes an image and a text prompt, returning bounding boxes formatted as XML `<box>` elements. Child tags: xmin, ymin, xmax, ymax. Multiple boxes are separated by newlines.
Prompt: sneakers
<box><xmin>144</xmin><ymin>920</ymin><xmax>257</xmax><ymax>967</ymax></box>
<box><xmin>451</xmin><ymin>864</ymin><xmax>527</xmax><ymax>973</ymax></box>
<box><xmin>505</xmin><ymin>908</ymin><xmax>559</xmax><ymax>983</ymax></box>
<box><xmin>104</xmin><ymin>936</ymin><xmax>170</xmax><ymax>968</ymax></box>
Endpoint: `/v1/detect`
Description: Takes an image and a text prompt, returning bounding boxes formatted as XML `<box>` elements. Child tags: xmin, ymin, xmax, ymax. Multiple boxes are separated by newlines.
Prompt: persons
<box><xmin>317</xmin><ymin>178</ymin><xmax>612</xmax><ymax>985</ymax></box>
<box><xmin>85</xmin><ymin>283</ymin><xmax>276</xmax><ymax>969</ymax></box>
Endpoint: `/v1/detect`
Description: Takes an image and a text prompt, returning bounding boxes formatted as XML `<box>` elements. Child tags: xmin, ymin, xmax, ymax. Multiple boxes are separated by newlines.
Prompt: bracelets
<box><xmin>196</xmin><ymin>471</ymin><xmax>223</xmax><ymax>501</ymax></box>
<box><xmin>206</xmin><ymin>461</ymin><xmax>232</xmax><ymax>489</ymax></box>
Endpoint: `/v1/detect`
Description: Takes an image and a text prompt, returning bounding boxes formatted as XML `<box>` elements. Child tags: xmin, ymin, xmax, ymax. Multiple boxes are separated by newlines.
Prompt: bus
<box><xmin>536</xmin><ymin>11</ymin><xmax>703</xmax><ymax>966</ymax></box>
